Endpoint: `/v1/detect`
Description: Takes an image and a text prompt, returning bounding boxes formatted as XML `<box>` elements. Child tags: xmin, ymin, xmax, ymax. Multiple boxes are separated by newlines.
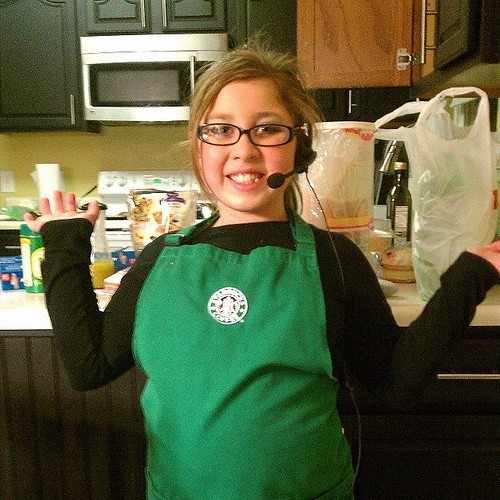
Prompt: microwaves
<box><xmin>80</xmin><ymin>33</ymin><xmax>231</xmax><ymax>125</ymax></box>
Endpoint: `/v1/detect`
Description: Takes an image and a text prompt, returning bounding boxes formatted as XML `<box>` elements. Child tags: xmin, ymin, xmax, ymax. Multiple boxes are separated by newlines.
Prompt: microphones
<box><xmin>267</xmin><ymin>133</ymin><xmax>316</xmax><ymax>188</ymax></box>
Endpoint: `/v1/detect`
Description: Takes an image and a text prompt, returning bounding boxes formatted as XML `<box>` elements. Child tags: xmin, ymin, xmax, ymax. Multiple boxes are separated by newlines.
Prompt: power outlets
<box><xmin>0</xmin><ymin>170</ymin><xmax>16</xmax><ymax>192</ymax></box>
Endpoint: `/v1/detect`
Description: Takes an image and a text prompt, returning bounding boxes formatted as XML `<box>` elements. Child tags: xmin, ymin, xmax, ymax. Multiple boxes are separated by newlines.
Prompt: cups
<box><xmin>19</xmin><ymin>224</ymin><xmax>46</xmax><ymax>293</ymax></box>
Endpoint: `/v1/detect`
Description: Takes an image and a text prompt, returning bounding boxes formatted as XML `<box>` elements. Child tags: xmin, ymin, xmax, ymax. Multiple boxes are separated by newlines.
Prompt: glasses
<box><xmin>196</xmin><ymin>122</ymin><xmax>301</xmax><ymax>148</ymax></box>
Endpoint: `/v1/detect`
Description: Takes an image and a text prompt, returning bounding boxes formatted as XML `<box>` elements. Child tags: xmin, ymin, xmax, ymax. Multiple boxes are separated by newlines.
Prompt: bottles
<box><xmin>386</xmin><ymin>161</ymin><xmax>413</xmax><ymax>246</ymax></box>
<box><xmin>81</xmin><ymin>201</ymin><xmax>114</xmax><ymax>289</ymax></box>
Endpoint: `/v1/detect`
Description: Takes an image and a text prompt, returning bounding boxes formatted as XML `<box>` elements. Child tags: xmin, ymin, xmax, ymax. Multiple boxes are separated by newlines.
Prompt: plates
<box><xmin>379</xmin><ymin>278</ymin><xmax>399</xmax><ymax>297</ymax></box>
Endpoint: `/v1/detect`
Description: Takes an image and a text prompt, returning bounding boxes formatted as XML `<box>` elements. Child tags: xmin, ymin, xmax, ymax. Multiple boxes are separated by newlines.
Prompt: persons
<box><xmin>23</xmin><ymin>43</ymin><xmax>499</xmax><ymax>500</ymax></box>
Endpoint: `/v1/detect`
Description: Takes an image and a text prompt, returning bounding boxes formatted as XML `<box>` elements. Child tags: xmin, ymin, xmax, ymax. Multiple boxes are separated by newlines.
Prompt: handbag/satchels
<box><xmin>371</xmin><ymin>87</ymin><xmax>500</xmax><ymax>301</ymax></box>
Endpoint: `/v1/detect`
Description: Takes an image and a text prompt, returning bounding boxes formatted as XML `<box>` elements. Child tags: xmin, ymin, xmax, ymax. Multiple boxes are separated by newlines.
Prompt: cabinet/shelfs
<box><xmin>0</xmin><ymin>0</ymin><xmax>500</xmax><ymax>134</ymax></box>
<box><xmin>0</xmin><ymin>326</ymin><xmax>500</xmax><ymax>500</ymax></box>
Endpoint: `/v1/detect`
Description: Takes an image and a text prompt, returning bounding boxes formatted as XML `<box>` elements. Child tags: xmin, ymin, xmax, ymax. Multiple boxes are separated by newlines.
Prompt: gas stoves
<box><xmin>90</xmin><ymin>169</ymin><xmax>218</xmax><ymax>230</ymax></box>
